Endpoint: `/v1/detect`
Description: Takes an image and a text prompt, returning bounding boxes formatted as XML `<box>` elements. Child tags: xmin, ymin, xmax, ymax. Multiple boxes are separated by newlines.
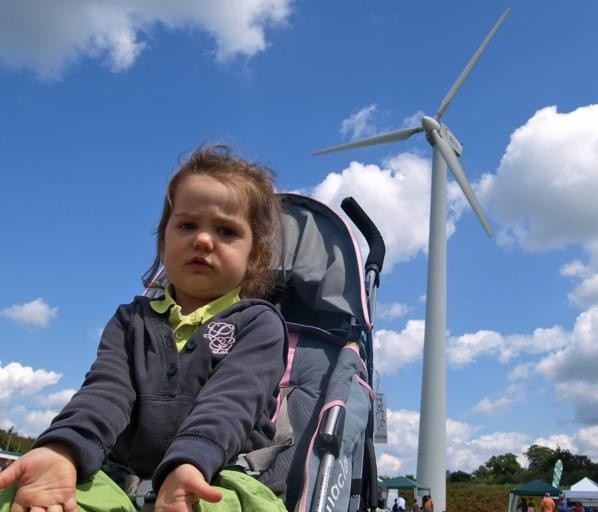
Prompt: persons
<box><xmin>527</xmin><ymin>497</ymin><xmax>535</xmax><ymax>512</ymax></box>
<box><xmin>392</xmin><ymin>498</ymin><xmax>399</xmax><ymax>512</ymax></box>
<box><xmin>395</xmin><ymin>494</ymin><xmax>406</xmax><ymax>511</ymax></box>
<box><xmin>558</xmin><ymin>495</ymin><xmax>568</xmax><ymax>511</ymax></box>
<box><xmin>411</xmin><ymin>498</ymin><xmax>420</xmax><ymax>512</ymax></box>
<box><xmin>0</xmin><ymin>141</ymin><xmax>291</xmax><ymax>512</ymax></box>
<box><xmin>423</xmin><ymin>495</ymin><xmax>433</xmax><ymax>512</ymax></box>
<box><xmin>516</xmin><ymin>498</ymin><xmax>528</xmax><ymax>511</ymax></box>
<box><xmin>573</xmin><ymin>502</ymin><xmax>585</xmax><ymax>512</ymax></box>
<box><xmin>541</xmin><ymin>491</ymin><xmax>556</xmax><ymax>512</ymax></box>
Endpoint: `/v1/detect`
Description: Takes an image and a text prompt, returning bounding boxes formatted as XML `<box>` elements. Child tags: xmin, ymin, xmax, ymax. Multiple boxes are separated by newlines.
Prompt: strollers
<box><xmin>144</xmin><ymin>194</ymin><xmax>386</xmax><ymax>512</ymax></box>
